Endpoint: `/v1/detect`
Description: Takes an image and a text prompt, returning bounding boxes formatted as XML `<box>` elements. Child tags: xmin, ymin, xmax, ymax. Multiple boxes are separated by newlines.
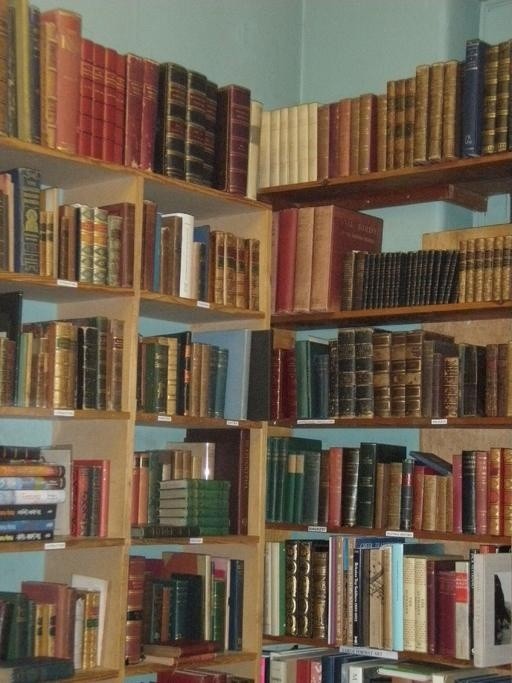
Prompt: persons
<box><xmin>0</xmin><ymin>574</ymin><xmax>108</xmax><ymax>683</ymax></box>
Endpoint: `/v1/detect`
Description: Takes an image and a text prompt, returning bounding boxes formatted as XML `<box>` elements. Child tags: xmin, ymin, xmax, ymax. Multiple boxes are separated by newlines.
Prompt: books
<box><xmin>1</xmin><ymin>292</ymin><xmax>124</xmax><ymax>411</ymax></box>
<box><xmin>267</xmin><ymin>436</ymin><xmax>512</xmax><ymax>535</ymax></box>
<box><xmin>270</xmin><ymin>327</ymin><xmax>512</xmax><ymax>420</ymax></box>
<box><xmin>132</xmin><ymin>428</ymin><xmax>250</xmax><ymax>539</ymax></box>
<box><xmin>271</xmin><ymin>205</ymin><xmax>512</xmax><ymax>315</ymax></box>
<box><xmin>142</xmin><ymin>199</ymin><xmax>260</xmax><ymax>312</ymax></box>
<box><xmin>0</xmin><ymin>167</ymin><xmax>134</xmax><ymax>288</ymax></box>
<box><xmin>0</xmin><ymin>0</ymin><xmax>512</xmax><ymax>201</ymax></box>
<box><xmin>0</xmin><ymin>444</ymin><xmax>111</xmax><ymax>541</ymax></box>
<box><xmin>124</xmin><ymin>551</ymin><xmax>245</xmax><ymax>665</ymax></box>
<box><xmin>157</xmin><ymin>666</ymin><xmax>254</xmax><ymax>683</ymax></box>
<box><xmin>138</xmin><ymin>331</ymin><xmax>229</xmax><ymax>418</ymax></box>
<box><xmin>259</xmin><ymin>535</ymin><xmax>512</xmax><ymax>683</ymax></box>
